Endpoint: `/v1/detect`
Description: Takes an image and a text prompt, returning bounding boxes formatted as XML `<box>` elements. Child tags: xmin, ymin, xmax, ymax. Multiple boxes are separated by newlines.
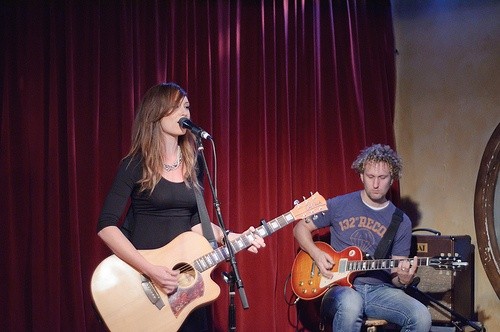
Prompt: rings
<box><xmin>401</xmin><ymin>267</ymin><xmax>406</xmax><ymax>271</ymax></box>
<box><xmin>404</xmin><ymin>268</ymin><xmax>408</xmax><ymax>272</ymax></box>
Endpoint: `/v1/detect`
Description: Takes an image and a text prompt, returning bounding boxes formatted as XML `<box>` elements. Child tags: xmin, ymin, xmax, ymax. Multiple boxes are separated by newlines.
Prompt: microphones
<box><xmin>179</xmin><ymin>117</ymin><xmax>212</xmax><ymax>141</ymax></box>
<box><xmin>407</xmin><ymin>276</ymin><xmax>420</xmax><ymax>290</ymax></box>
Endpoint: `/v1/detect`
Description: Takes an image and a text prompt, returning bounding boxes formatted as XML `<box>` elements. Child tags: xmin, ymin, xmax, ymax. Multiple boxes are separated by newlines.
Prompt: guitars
<box><xmin>291</xmin><ymin>241</ymin><xmax>463</xmax><ymax>301</ymax></box>
<box><xmin>90</xmin><ymin>191</ymin><xmax>328</xmax><ymax>332</ymax></box>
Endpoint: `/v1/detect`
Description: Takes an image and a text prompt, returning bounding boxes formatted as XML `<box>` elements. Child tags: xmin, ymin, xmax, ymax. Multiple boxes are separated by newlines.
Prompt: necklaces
<box><xmin>163</xmin><ymin>145</ymin><xmax>182</xmax><ymax>171</ymax></box>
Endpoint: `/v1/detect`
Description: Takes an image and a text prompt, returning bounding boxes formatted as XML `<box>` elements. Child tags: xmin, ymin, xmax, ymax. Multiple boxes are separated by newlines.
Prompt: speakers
<box><xmin>403</xmin><ymin>234</ymin><xmax>472</xmax><ymax>326</ymax></box>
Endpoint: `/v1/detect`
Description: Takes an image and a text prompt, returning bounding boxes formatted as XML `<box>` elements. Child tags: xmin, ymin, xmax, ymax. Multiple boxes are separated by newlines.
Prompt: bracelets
<box><xmin>221</xmin><ymin>229</ymin><xmax>230</xmax><ymax>246</ymax></box>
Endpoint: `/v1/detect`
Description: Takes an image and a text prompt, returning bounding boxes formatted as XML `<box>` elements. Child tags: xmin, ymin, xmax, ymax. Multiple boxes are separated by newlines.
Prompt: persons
<box><xmin>95</xmin><ymin>83</ymin><xmax>266</xmax><ymax>332</ymax></box>
<box><xmin>293</xmin><ymin>143</ymin><xmax>432</xmax><ymax>332</ymax></box>
